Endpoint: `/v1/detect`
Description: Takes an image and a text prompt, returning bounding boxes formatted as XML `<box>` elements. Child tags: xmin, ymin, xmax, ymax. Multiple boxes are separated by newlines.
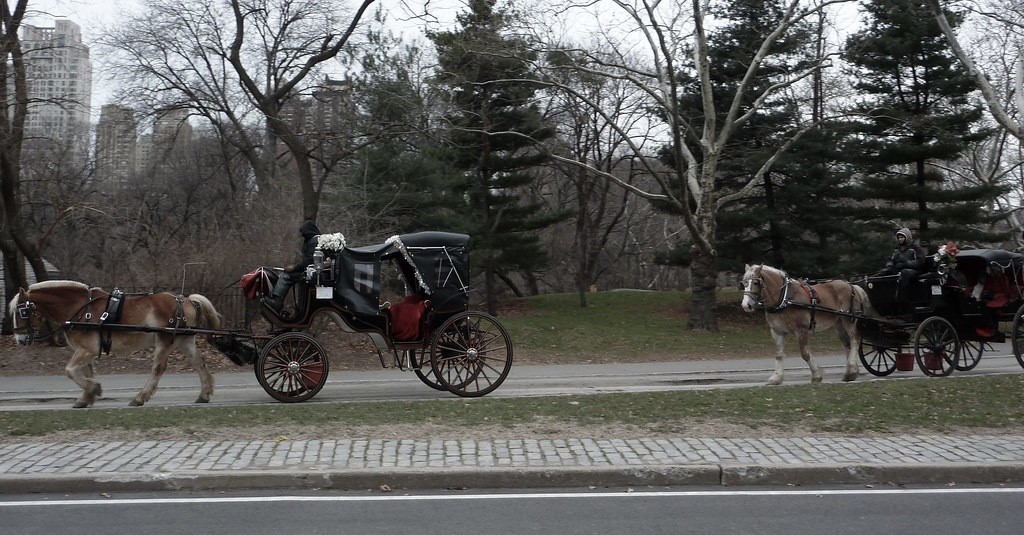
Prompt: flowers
<box><xmin>934</xmin><ymin>241</ymin><xmax>961</xmax><ymax>275</ymax></box>
<box><xmin>316</xmin><ymin>232</ymin><xmax>347</xmax><ymax>253</ymax></box>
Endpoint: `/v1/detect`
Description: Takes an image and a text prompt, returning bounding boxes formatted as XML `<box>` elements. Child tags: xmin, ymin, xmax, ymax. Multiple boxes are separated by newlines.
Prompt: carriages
<box><xmin>10</xmin><ymin>232</ymin><xmax>515</xmax><ymax>409</ymax></box>
<box><xmin>742</xmin><ymin>248</ymin><xmax>1023</xmax><ymax>386</ymax></box>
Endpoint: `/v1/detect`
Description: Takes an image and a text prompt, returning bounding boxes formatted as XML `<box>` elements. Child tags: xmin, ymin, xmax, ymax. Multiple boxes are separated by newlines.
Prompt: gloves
<box><xmin>283</xmin><ymin>265</ymin><xmax>294</xmax><ymax>274</ymax></box>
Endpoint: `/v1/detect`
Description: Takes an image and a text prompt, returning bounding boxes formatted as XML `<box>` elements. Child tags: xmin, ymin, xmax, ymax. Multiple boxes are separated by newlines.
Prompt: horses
<box><xmin>741</xmin><ymin>262</ymin><xmax>871</xmax><ymax>385</ymax></box>
<box><xmin>8</xmin><ymin>280</ymin><xmax>222</xmax><ymax>407</ymax></box>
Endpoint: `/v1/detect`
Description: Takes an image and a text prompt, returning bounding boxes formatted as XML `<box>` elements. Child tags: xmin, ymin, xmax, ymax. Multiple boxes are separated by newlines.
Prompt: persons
<box><xmin>264</xmin><ymin>220</ymin><xmax>334</xmax><ymax>315</ymax></box>
<box><xmin>879</xmin><ymin>227</ymin><xmax>926</xmax><ymax>283</ymax></box>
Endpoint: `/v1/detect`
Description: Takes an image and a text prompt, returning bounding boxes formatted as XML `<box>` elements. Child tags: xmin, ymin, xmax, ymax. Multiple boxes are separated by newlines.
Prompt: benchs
<box><xmin>964</xmin><ymin>274</ymin><xmax>1023</xmax><ymax>307</ymax></box>
<box><xmin>868</xmin><ymin>255</ymin><xmax>938</xmax><ymax>302</ymax></box>
<box><xmin>300</xmin><ymin>255</ymin><xmax>336</xmax><ymax>284</ymax></box>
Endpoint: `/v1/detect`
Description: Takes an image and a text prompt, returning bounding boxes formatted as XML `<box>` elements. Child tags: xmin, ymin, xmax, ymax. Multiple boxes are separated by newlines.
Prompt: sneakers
<box><xmin>263</xmin><ymin>295</ymin><xmax>284</xmax><ymax>315</ymax></box>
